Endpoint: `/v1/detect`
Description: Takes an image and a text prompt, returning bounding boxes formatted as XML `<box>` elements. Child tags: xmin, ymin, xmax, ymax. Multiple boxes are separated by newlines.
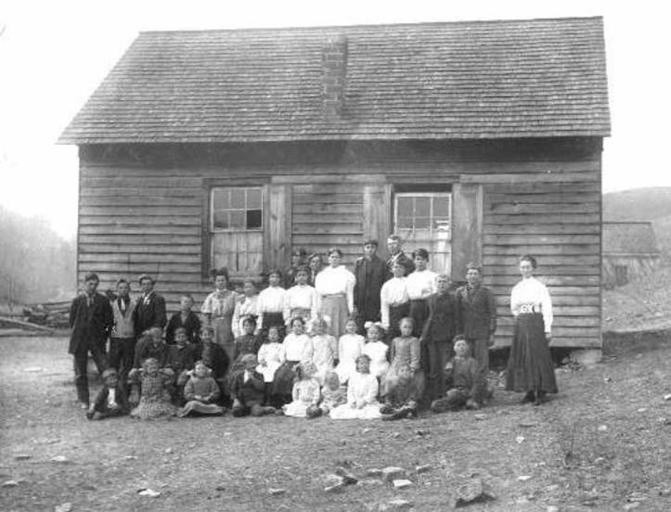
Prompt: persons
<box><xmin>86</xmin><ymin>232</ymin><xmax>502</xmax><ymax>419</ymax></box>
<box><xmin>507</xmin><ymin>255</ymin><xmax>556</xmax><ymax>405</ymax></box>
<box><xmin>66</xmin><ymin>273</ymin><xmax>113</xmax><ymax>409</ymax></box>
